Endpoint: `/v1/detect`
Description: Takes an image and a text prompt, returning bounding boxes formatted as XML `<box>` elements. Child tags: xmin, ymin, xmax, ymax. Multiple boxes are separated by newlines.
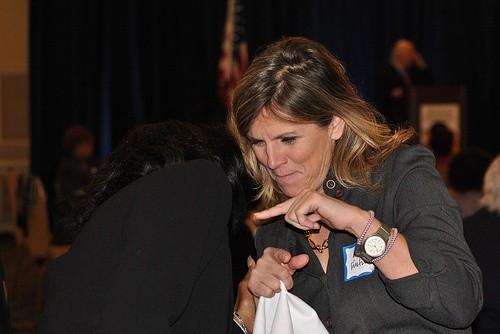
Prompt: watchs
<box><xmin>359</xmin><ymin>223</ymin><xmax>391</xmax><ymax>261</ymax></box>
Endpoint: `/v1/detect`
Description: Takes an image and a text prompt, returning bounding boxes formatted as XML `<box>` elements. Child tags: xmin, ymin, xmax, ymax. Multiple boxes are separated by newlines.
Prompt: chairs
<box><xmin>7</xmin><ymin>172</ymin><xmax>29</xmax><ymax>302</ymax></box>
<box><xmin>25</xmin><ymin>175</ymin><xmax>71</xmax><ymax>309</ymax></box>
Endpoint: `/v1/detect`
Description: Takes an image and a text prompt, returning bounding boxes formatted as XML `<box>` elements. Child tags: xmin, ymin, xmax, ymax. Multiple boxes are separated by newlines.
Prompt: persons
<box><xmin>47</xmin><ymin>127</ymin><xmax>106</xmax><ymax>245</ymax></box>
<box><xmin>366</xmin><ymin>39</ymin><xmax>500</xmax><ymax>333</ymax></box>
<box><xmin>38</xmin><ymin>119</ymin><xmax>260</xmax><ymax>334</ymax></box>
<box><xmin>231</xmin><ymin>35</ymin><xmax>484</xmax><ymax>334</ymax></box>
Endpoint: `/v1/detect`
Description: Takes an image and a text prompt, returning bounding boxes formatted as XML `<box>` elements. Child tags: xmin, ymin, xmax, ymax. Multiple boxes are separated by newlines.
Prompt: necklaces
<box><xmin>304</xmin><ymin>229</ymin><xmax>329</xmax><ymax>253</ymax></box>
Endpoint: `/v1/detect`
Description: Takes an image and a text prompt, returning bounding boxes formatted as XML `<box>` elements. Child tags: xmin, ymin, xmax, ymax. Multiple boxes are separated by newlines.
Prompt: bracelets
<box><xmin>365</xmin><ymin>228</ymin><xmax>398</xmax><ymax>263</ymax></box>
<box><xmin>351</xmin><ymin>210</ymin><xmax>375</xmax><ymax>255</ymax></box>
<box><xmin>233</xmin><ymin>311</ymin><xmax>252</xmax><ymax>334</ymax></box>
